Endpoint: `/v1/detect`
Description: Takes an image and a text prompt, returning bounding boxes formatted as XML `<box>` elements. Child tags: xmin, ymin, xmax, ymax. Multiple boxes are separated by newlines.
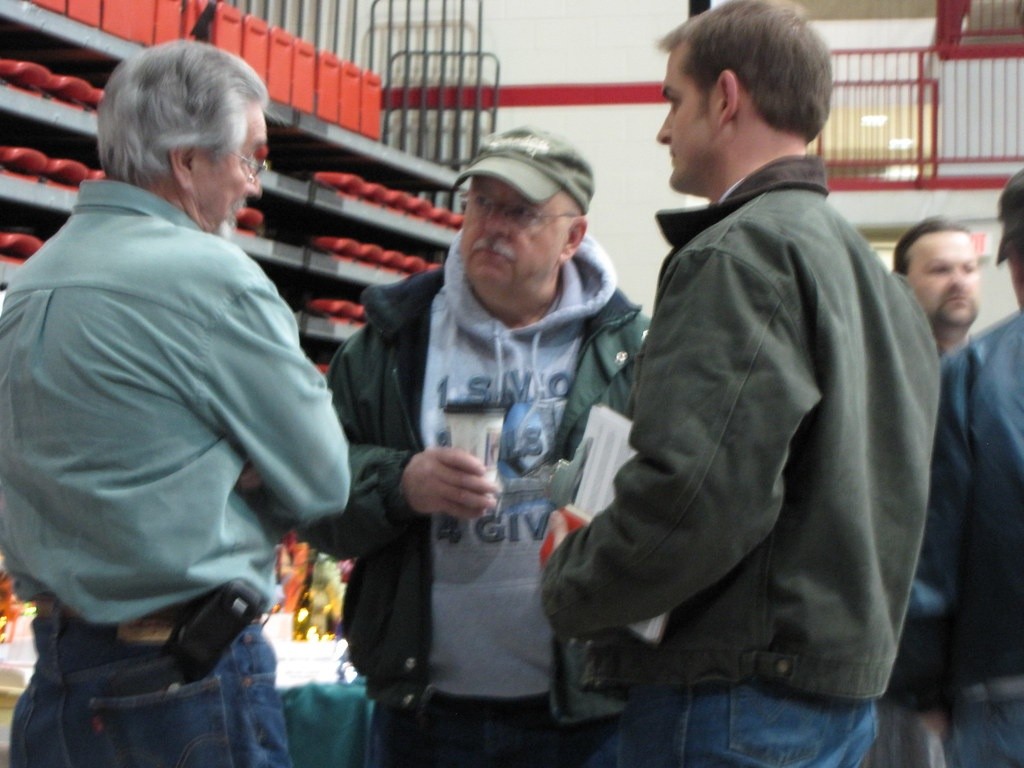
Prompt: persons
<box><xmin>297</xmin><ymin>125</ymin><xmax>651</xmax><ymax>768</ymax></box>
<box><xmin>540</xmin><ymin>0</ymin><xmax>941</xmax><ymax>768</ymax></box>
<box><xmin>0</xmin><ymin>41</ymin><xmax>352</xmax><ymax>768</ymax></box>
<box><xmin>883</xmin><ymin>170</ymin><xmax>1024</xmax><ymax>768</ymax></box>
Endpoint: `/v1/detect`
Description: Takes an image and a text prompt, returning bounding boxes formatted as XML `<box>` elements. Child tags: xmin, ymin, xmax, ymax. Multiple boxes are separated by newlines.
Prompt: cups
<box><xmin>440</xmin><ymin>395</ymin><xmax>515</xmax><ymax>486</ymax></box>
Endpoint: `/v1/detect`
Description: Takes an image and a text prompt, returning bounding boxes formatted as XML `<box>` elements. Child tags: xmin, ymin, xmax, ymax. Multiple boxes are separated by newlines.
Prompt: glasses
<box><xmin>460</xmin><ymin>193</ymin><xmax>582</xmax><ymax>227</ymax></box>
<box><xmin>219</xmin><ymin>145</ymin><xmax>267</xmax><ymax>182</ymax></box>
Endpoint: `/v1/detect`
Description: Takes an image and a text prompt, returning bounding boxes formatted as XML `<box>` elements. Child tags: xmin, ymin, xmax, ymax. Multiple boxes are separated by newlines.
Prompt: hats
<box><xmin>455</xmin><ymin>127</ymin><xmax>594</xmax><ymax>216</ymax></box>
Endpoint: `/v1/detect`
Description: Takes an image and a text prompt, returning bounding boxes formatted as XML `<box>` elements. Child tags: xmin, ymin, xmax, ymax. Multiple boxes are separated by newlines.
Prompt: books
<box><xmin>540</xmin><ymin>405</ymin><xmax>665</xmax><ymax>640</ymax></box>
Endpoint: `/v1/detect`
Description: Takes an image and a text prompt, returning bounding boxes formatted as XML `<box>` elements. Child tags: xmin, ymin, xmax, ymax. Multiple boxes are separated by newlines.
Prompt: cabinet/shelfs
<box><xmin>0</xmin><ymin>1</ymin><xmax>468</xmax><ymax>343</ymax></box>
